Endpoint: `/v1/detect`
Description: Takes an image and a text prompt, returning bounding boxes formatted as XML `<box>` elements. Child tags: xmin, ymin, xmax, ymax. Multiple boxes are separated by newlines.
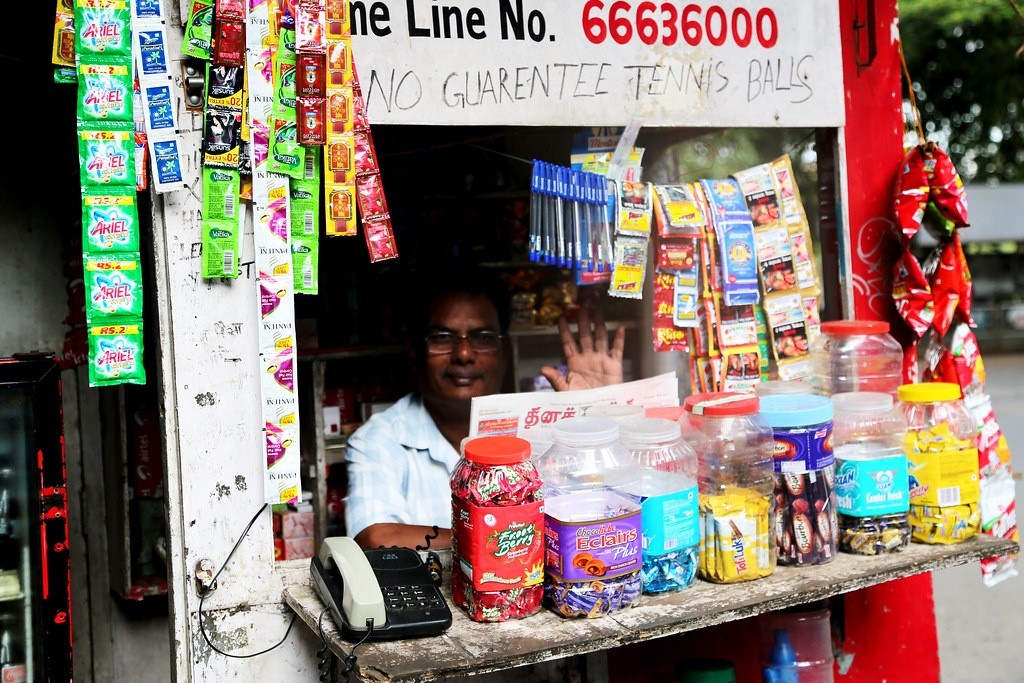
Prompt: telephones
<box><xmin>310</xmin><ymin>537</ymin><xmax>453</xmax><ymax>643</ymax></box>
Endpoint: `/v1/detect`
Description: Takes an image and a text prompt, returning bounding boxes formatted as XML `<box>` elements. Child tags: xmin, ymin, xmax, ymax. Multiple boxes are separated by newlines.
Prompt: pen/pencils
<box><xmin>529</xmin><ymin>159</ymin><xmax>614</xmax><ymax>272</ymax></box>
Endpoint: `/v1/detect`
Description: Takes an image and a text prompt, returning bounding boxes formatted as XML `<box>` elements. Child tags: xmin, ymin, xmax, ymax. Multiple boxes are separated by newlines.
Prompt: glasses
<box><xmin>425</xmin><ymin>332</ymin><xmax>502</xmax><ymax>353</ymax></box>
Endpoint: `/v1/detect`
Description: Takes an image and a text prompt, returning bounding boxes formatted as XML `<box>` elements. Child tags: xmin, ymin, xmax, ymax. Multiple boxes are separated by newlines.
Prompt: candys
<box><xmin>449</xmin><ymin>462</ymin><xmax>543</xmax><ymax>623</ymax></box>
<box><xmin>539</xmin><ymin>417</ymin><xmax>976</xmax><ymax>620</ymax></box>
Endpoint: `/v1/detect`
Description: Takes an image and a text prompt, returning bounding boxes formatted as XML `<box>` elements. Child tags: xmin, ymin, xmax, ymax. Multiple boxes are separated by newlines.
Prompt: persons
<box><xmin>344</xmin><ymin>282</ymin><xmax>625</xmax><ymax>553</ymax></box>
